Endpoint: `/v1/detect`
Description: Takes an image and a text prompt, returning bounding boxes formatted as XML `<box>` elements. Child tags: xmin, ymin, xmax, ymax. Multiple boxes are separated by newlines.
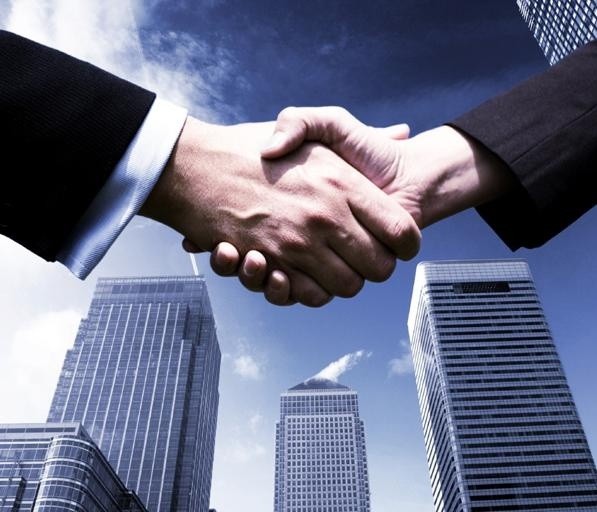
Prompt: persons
<box><xmin>182</xmin><ymin>37</ymin><xmax>597</xmax><ymax>304</ymax></box>
<box><xmin>0</xmin><ymin>29</ymin><xmax>421</xmax><ymax>307</ymax></box>
